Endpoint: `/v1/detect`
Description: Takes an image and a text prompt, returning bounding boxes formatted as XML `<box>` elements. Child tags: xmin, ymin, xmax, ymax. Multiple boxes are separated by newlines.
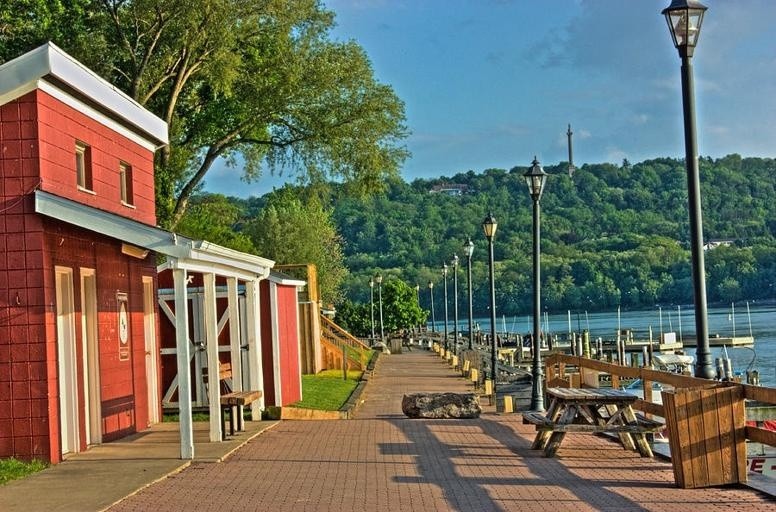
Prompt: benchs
<box><xmin>202</xmin><ymin>363</ymin><xmax>262</xmax><ymax>441</ymax></box>
<box><xmin>522</xmin><ymin>413</ymin><xmax>664</xmax><ymax>434</ymax></box>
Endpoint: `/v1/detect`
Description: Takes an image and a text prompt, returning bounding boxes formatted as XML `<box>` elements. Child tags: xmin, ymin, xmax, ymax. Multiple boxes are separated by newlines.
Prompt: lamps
<box><xmin>121</xmin><ymin>243</ymin><xmax>149</xmax><ymax>259</ymax></box>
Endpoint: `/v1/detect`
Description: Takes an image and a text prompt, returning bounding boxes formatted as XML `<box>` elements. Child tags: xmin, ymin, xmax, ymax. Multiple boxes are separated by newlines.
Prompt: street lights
<box><xmin>415</xmin><ymin>280</ymin><xmax>437</xmax><ymax>337</ymax></box>
<box><xmin>524</xmin><ymin>156</ymin><xmax>549</xmax><ymax>412</ymax></box>
<box><xmin>662</xmin><ymin>1</ymin><xmax>720</xmax><ymax>380</ymax></box>
<box><xmin>369</xmin><ymin>272</ymin><xmax>385</xmax><ymax>352</ymax></box>
<box><xmin>441</xmin><ymin>236</ymin><xmax>475</xmax><ymax>355</ymax></box>
<box><xmin>483</xmin><ymin>210</ymin><xmax>498</xmax><ymax>393</ymax></box>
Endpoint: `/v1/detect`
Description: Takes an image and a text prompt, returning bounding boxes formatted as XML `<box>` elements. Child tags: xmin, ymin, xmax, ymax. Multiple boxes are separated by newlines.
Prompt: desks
<box><xmin>531</xmin><ymin>387</ymin><xmax>654</xmax><ymax>458</ymax></box>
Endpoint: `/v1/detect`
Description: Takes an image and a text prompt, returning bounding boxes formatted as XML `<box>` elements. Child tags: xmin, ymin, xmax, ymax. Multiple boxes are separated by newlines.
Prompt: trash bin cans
<box><xmin>390</xmin><ymin>337</ymin><xmax>403</xmax><ymax>354</ymax></box>
